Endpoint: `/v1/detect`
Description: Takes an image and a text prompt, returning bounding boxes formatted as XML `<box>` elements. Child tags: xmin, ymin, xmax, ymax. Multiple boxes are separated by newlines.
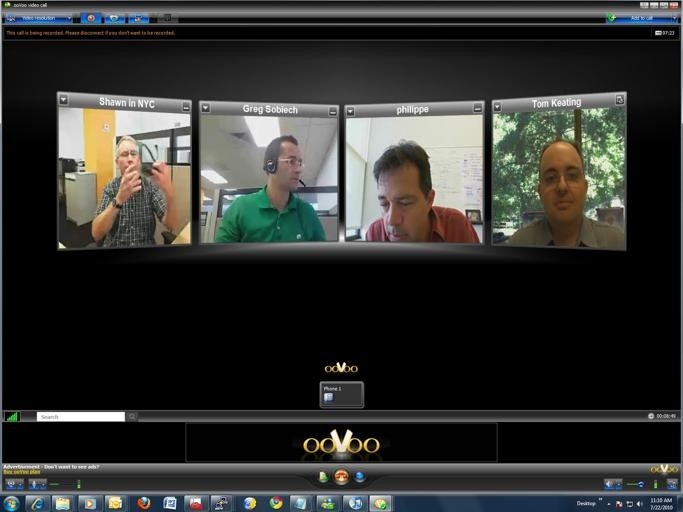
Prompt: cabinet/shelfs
<box><xmin>64</xmin><ymin>172</ymin><xmax>97</xmax><ymax>226</ymax></box>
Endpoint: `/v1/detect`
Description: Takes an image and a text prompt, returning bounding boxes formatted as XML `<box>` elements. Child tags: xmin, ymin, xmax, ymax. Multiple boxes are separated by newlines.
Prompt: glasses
<box><xmin>278</xmin><ymin>156</ymin><xmax>306</xmax><ymax>169</ymax></box>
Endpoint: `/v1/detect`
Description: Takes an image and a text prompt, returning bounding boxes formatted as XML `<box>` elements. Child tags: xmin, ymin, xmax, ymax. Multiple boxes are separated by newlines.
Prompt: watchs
<box><xmin>111</xmin><ymin>197</ymin><xmax>122</xmax><ymax>210</ymax></box>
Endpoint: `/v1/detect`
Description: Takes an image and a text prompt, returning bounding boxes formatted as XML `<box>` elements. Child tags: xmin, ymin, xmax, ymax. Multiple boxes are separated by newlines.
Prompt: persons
<box><xmin>504</xmin><ymin>140</ymin><xmax>625</xmax><ymax>251</ymax></box>
<box><xmin>210</xmin><ymin>132</ymin><xmax>326</xmax><ymax>242</ymax></box>
<box><xmin>365</xmin><ymin>138</ymin><xmax>480</xmax><ymax>243</ymax></box>
<box><xmin>88</xmin><ymin>135</ymin><xmax>180</xmax><ymax>247</ymax></box>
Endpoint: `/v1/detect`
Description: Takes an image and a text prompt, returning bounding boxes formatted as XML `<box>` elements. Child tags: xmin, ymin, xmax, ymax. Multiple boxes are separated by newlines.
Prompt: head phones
<box><xmin>265</xmin><ymin>139</ymin><xmax>276</xmax><ymax>173</ymax></box>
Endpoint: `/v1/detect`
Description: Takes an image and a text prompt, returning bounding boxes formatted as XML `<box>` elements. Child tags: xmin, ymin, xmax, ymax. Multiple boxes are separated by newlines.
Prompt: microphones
<box><xmin>298</xmin><ymin>179</ymin><xmax>306</xmax><ymax>187</ymax></box>
